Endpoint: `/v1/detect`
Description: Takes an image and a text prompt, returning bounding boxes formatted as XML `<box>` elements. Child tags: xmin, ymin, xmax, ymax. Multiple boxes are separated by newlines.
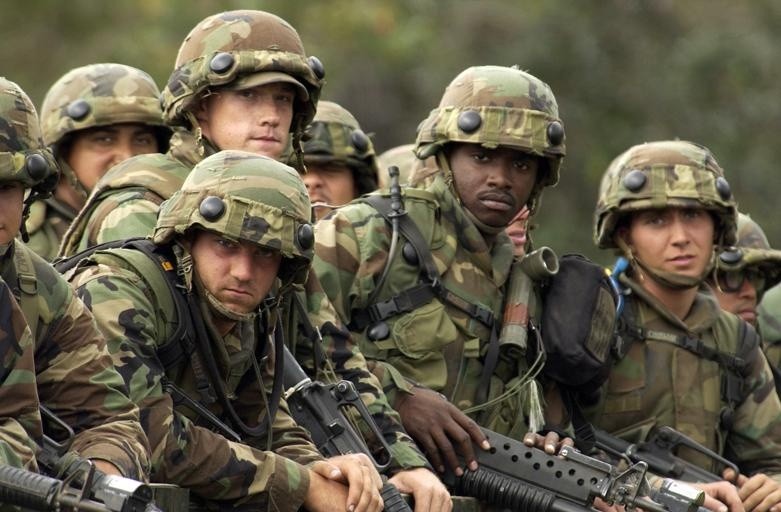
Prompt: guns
<box><xmin>277</xmin><ymin>338</ymin><xmax>412</xmax><ymax>512</ymax></box>
<box><xmin>572</xmin><ymin>415</ymin><xmax>738</xmax><ymax>495</ymax></box>
<box><xmin>427</xmin><ymin>413</ymin><xmax>722</xmax><ymax>512</ymax></box>
<box><xmin>0</xmin><ymin>455</ymin><xmax>151</xmax><ymax>512</ymax></box>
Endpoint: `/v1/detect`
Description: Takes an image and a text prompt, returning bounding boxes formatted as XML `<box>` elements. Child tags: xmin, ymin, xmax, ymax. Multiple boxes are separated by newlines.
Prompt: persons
<box><xmin>43</xmin><ymin>150</ymin><xmax>385</xmax><ymax>512</ymax></box>
<box><xmin>312</xmin><ymin>65</ymin><xmax>564</xmax><ymax>511</ymax></box>
<box><xmin>52</xmin><ymin>9</ymin><xmax>325</xmax><ymax>261</ymax></box>
<box><xmin>369</xmin><ymin>140</ymin><xmax>433</xmax><ymax>195</ymax></box>
<box><xmin>19</xmin><ymin>63</ymin><xmax>167</xmax><ymax>263</ymax></box>
<box><xmin>755</xmin><ymin>285</ymin><xmax>781</xmax><ymax>373</ymax></box>
<box><xmin>693</xmin><ymin>210</ymin><xmax>778</xmax><ymax>333</ymax></box>
<box><xmin>582</xmin><ymin>140</ymin><xmax>780</xmax><ymax>512</ymax></box>
<box><xmin>292</xmin><ymin>97</ymin><xmax>379</xmax><ymax>230</ymax></box>
<box><xmin>1</xmin><ymin>77</ymin><xmax>154</xmax><ymax>512</ymax></box>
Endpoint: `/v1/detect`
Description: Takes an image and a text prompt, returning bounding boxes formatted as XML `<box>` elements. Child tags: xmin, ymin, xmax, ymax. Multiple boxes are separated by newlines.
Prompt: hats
<box><xmin>151</xmin><ymin>150</ymin><xmax>314</xmax><ymax>323</ymax></box>
<box><xmin>0</xmin><ymin>75</ymin><xmax>59</xmax><ymax>259</ymax></box>
<box><xmin>36</xmin><ymin>61</ymin><xmax>171</xmax><ymax>205</ymax></box>
<box><xmin>694</xmin><ymin>212</ymin><xmax>781</xmax><ymax>290</ymax></box>
<box><xmin>413</xmin><ymin>64</ymin><xmax>567</xmax><ymax>235</ymax></box>
<box><xmin>159</xmin><ymin>7</ymin><xmax>327</xmax><ymax>175</ymax></box>
<box><xmin>295</xmin><ymin>100</ymin><xmax>382</xmax><ymax>194</ymax></box>
<box><xmin>593</xmin><ymin>140</ymin><xmax>740</xmax><ymax>291</ymax></box>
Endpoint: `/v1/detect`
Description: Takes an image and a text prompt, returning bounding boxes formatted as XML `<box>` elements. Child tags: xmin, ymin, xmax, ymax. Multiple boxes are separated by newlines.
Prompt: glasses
<box><xmin>722</xmin><ymin>269</ymin><xmax>768</xmax><ymax>291</ymax></box>
